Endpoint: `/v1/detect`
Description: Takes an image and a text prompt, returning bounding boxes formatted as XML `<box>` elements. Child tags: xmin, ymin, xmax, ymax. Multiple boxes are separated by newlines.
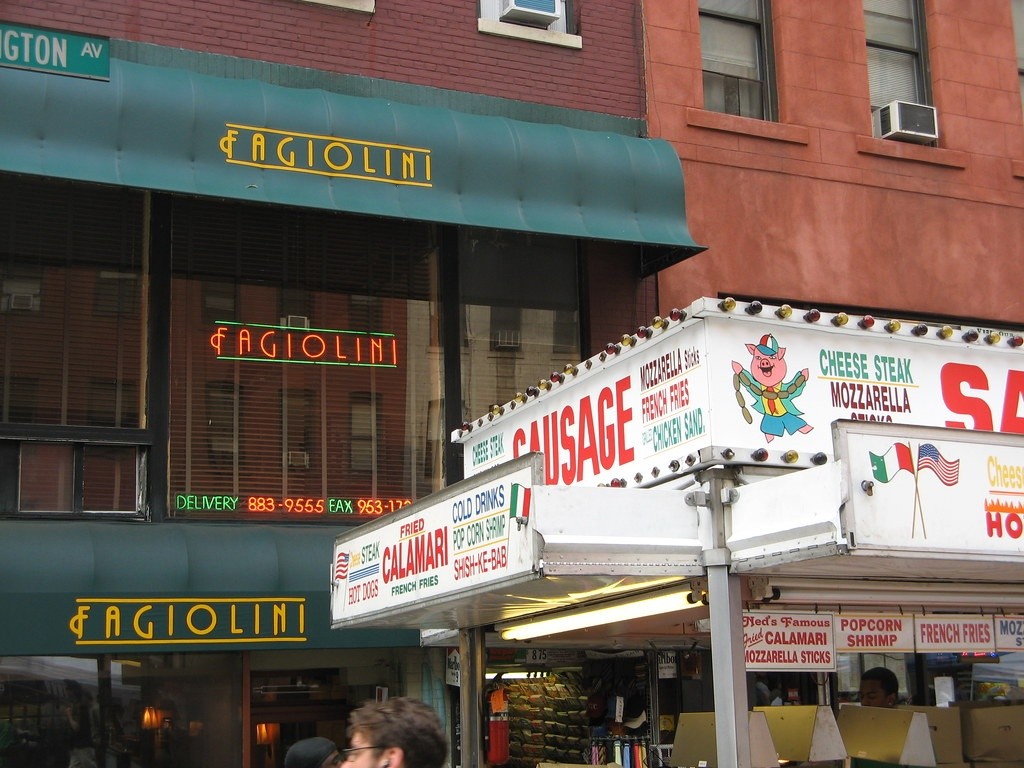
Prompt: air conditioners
<box><xmin>287</xmin><ymin>315</ymin><xmax>308</xmax><ymax>332</ymax></box>
<box><xmin>872</xmin><ymin>100</ymin><xmax>938</xmax><ymax>145</ymax></box>
<box><xmin>11</xmin><ymin>293</ymin><xmax>34</xmax><ymax>310</ymax></box>
<box><xmin>499</xmin><ymin>0</ymin><xmax>561</xmax><ymax>29</ymax></box>
<box><xmin>495</xmin><ymin>330</ymin><xmax>519</xmax><ymax>349</ymax></box>
<box><xmin>288</xmin><ymin>450</ymin><xmax>307</xmax><ymax>467</ymax></box>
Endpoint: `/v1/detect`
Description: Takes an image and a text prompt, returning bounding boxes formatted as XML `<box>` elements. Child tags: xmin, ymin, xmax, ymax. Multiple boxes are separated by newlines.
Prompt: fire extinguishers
<box><xmin>482</xmin><ymin>671</ymin><xmax>512</xmax><ymax>766</ymax></box>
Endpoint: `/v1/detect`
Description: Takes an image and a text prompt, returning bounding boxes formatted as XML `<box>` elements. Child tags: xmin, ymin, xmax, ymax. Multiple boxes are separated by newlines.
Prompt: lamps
<box><xmin>140</xmin><ymin>705</ymin><xmax>161</xmax><ymax>732</ymax></box>
<box><xmin>256</xmin><ymin>724</ymin><xmax>272</xmax><ymax>746</ymax></box>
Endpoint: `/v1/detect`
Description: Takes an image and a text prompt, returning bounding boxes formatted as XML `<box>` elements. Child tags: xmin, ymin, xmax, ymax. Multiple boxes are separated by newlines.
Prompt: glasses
<box><xmin>343</xmin><ymin>745</ymin><xmax>388</xmax><ymax>762</ymax></box>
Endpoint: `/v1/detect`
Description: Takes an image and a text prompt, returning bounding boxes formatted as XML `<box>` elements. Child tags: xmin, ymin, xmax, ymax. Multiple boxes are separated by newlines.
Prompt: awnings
<box><xmin>0</xmin><ymin>518</ymin><xmax>436</xmax><ymax>657</ymax></box>
<box><xmin>0</xmin><ymin>24</ymin><xmax>708</xmax><ymax>279</ymax></box>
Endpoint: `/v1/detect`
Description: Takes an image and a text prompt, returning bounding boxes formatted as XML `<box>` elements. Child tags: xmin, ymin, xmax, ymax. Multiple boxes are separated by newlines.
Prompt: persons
<box><xmin>859</xmin><ymin>666</ymin><xmax>899</xmax><ymax>707</ymax></box>
<box><xmin>284</xmin><ymin>736</ymin><xmax>344</xmax><ymax>768</ymax></box>
<box><xmin>340</xmin><ymin>696</ymin><xmax>447</xmax><ymax>768</ymax></box>
<box><xmin>757</xmin><ymin>676</ymin><xmax>791</xmax><ymax>706</ymax></box>
<box><xmin>58</xmin><ymin>678</ymin><xmax>101</xmax><ymax>768</ymax></box>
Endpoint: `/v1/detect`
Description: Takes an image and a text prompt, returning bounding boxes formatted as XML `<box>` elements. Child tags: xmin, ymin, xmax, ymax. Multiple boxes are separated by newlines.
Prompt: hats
<box><xmin>285</xmin><ymin>737</ymin><xmax>337</xmax><ymax>768</ymax></box>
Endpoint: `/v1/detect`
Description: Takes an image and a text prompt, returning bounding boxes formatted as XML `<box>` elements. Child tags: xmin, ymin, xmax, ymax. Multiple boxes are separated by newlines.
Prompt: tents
<box><xmin>970</xmin><ymin>653</ymin><xmax>1024</xmax><ymax>700</ymax></box>
